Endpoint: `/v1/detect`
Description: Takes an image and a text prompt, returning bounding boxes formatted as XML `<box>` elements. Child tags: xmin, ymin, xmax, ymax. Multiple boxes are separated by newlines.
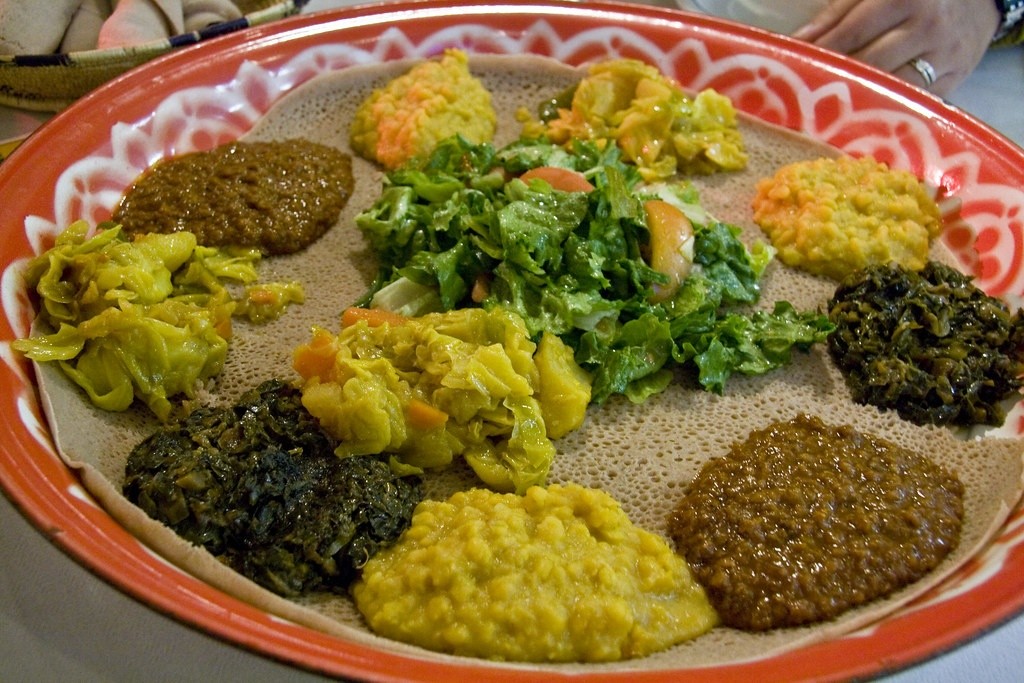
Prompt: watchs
<box><xmin>991</xmin><ymin>0</ymin><xmax>1024</xmax><ymax>42</ymax></box>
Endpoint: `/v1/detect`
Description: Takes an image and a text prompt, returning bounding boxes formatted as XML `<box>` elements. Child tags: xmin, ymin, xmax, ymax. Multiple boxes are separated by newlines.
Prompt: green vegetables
<box><xmin>348</xmin><ymin>117</ymin><xmax>844</xmax><ymax>406</ymax></box>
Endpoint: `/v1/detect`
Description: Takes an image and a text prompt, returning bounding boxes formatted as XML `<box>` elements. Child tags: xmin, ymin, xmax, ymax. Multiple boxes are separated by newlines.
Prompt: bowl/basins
<box><xmin>0</xmin><ymin>0</ymin><xmax>307</xmax><ymax>108</ymax></box>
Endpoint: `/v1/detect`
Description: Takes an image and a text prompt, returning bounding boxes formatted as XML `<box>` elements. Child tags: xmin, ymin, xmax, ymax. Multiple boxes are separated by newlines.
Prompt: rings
<box><xmin>909</xmin><ymin>56</ymin><xmax>937</xmax><ymax>86</ymax></box>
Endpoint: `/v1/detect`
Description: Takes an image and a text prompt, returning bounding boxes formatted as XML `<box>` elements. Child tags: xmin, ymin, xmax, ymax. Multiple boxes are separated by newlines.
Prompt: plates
<box><xmin>0</xmin><ymin>0</ymin><xmax>1023</xmax><ymax>683</ymax></box>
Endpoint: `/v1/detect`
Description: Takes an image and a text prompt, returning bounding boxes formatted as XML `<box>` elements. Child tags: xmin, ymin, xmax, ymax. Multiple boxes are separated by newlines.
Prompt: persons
<box><xmin>788</xmin><ymin>0</ymin><xmax>1024</xmax><ymax>103</ymax></box>
